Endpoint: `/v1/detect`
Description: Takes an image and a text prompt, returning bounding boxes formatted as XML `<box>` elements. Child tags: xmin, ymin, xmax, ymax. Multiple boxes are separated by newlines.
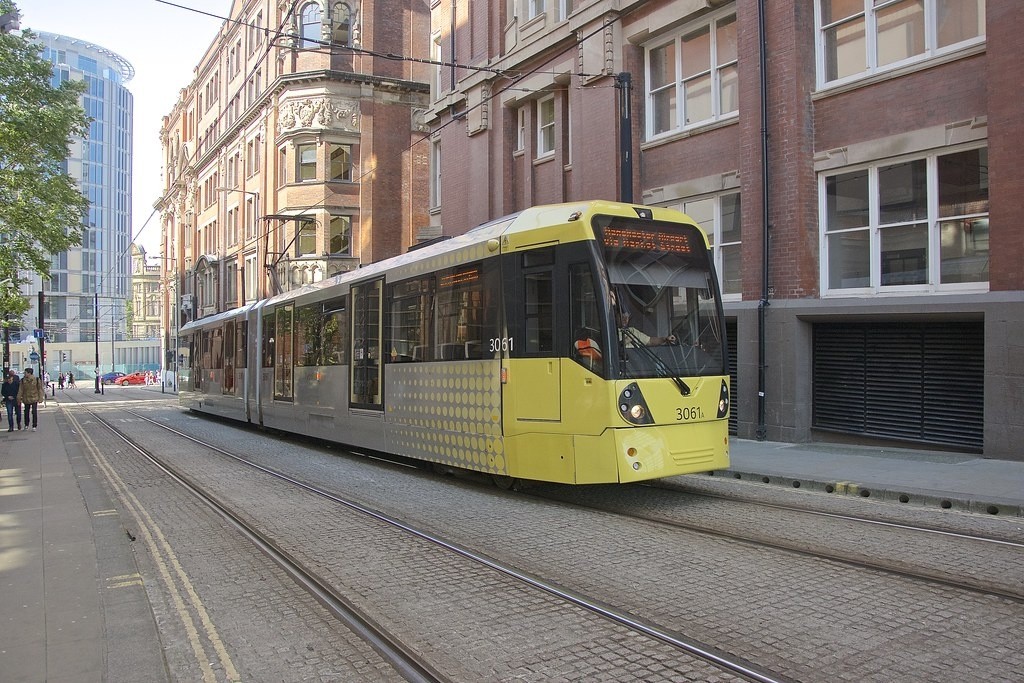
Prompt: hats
<box><xmin>5</xmin><ymin>373</ymin><xmax>11</xmax><ymax>378</ymax></box>
<box><xmin>614</xmin><ymin>304</ymin><xmax>627</xmax><ymax>313</ymax></box>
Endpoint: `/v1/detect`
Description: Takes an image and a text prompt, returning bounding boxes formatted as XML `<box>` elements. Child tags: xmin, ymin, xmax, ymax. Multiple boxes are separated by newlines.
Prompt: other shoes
<box><xmin>18</xmin><ymin>423</ymin><xmax>21</xmax><ymax>430</ymax></box>
<box><xmin>24</xmin><ymin>426</ymin><xmax>29</xmax><ymax>430</ymax></box>
<box><xmin>33</xmin><ymin>426</ymin><xmax>36</xmax><ymax>431</ymax></box>
<box><xmin>8</xmin><ymin>427</ymin><xmax>13</xmax><ymax>431</ymax></box>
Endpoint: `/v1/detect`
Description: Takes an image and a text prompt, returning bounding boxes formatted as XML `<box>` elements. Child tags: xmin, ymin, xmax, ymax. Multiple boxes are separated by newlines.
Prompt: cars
<box><xmin>101</xmin><ymin>371</ymin><xmax>127</xmax><ymax>386</ymax></box>
<box><xmin>114</xmin><ymin>372</ymin><xmax>156</xmax><ymax>386</ymax></box>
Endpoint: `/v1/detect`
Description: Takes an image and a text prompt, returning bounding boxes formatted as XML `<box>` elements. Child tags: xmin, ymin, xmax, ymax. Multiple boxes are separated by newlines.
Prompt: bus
<box><xmin>180</xmin><ymin>201</ymin><xmax>730</xmax><ymax>491</ymax></box>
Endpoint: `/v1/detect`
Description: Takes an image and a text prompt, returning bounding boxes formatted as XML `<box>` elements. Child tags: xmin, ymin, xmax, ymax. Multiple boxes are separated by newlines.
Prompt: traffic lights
<box><xmin>61</xmin><ymin>352</ymin><xmax>67</xmax><ymax>363</ymax></box>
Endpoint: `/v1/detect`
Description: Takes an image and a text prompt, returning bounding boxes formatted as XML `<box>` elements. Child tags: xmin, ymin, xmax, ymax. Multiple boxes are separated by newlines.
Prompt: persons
<box><xmin>616</xmin><ymin>310</ymin><xmax>676</xmax><ymax>348</ymax></box>
<box><xmin>0</xmin><ymin>367</ymin><xmax>77</xmax><ymax>432</ymax></box>
<box><xmin>144</xmin><ymin>370</ymin><xmax>160</xmax><ymax>385</ymax></box>
